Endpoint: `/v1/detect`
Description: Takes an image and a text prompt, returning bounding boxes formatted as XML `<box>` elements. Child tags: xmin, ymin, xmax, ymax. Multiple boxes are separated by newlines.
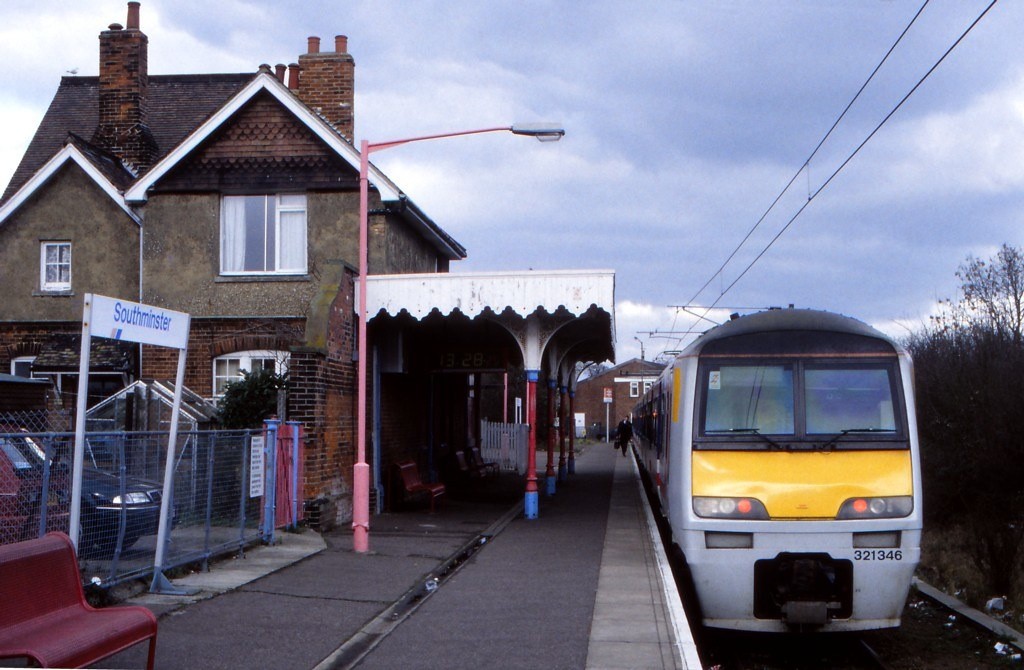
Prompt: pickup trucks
<box><xmin>586</xmin><ymin>421</ymin><xmax>618</xmax><ymax>443</ymax></box>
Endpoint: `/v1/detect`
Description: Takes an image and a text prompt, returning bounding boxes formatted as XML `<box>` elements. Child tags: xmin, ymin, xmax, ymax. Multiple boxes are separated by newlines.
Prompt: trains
<box><xmin>631</xmin><ymin>303</ymin><xmax>924</xmax><ymax>635</ymax></box>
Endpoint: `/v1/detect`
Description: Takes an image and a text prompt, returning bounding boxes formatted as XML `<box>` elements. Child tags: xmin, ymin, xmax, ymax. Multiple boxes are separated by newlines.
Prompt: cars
<box><xmin>0</xmin><ymin>424</ymin><xmax>182</xmax><ymax>554</ymax></box>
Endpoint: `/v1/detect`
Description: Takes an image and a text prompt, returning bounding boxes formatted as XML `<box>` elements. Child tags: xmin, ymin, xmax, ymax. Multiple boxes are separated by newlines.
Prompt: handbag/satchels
<box><xmin>614</xmin><ymin>438</ymin><xmax>620</xmax><ymax>448</ymax></box>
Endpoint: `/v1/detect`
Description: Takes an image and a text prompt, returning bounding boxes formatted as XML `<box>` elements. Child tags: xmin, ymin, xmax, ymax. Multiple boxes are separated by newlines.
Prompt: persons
<box><xmin>617</xmin><ymin>416</ymin><xmax>633</xmax><ymax>457</ymax></box>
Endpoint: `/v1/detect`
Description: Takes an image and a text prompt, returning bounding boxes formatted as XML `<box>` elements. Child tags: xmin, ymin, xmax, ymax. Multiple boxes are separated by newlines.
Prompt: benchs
<box><xmin>449</xmin><ymin>448</ymin><xmax>488</xmax><ymax>496</ymax></box>
<box><xmin>472</xmin><ymin>447</ymin><xmax>501</xmax><ymax>478</ymax></box>
<box><xmin>390</xmin><ymin>458</ymin><xmax>450</xmax><ymax>515</ymax></box>
<box><xmin>0</xmin><ymin>532</ymin><xmax>161</xmax><ymax>668</ymax></box>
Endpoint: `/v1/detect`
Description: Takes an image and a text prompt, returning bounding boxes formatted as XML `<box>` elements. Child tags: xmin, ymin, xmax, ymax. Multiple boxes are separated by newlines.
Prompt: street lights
<box><xmin>634</xmin><ymin>336</ymin><xmax>644</xmax><ymax>359</ymax></box>
<box><xmin>350</xmin><ymin>120</ymin><xmax>566</xmax><ymax>553</ymax></box>
